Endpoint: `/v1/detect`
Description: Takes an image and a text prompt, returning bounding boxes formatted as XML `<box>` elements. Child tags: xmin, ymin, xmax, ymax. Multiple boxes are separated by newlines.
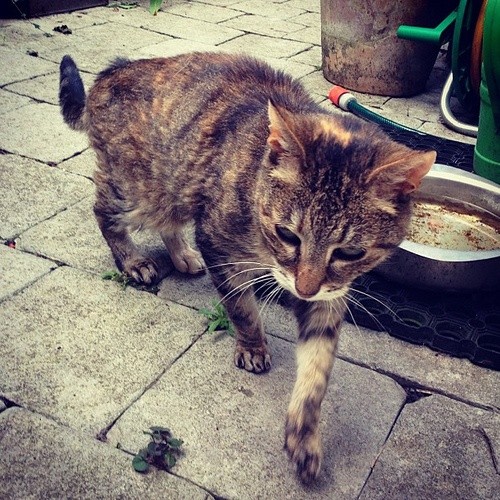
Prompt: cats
<box><xmin>59</xmin><ymin>53</ymin><xmax>437</xmax><ymax>489</ymax></box>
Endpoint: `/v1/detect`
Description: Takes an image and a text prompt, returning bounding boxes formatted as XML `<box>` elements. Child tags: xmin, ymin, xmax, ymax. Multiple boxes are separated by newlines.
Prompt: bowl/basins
<box><xmin>354</xmin><ymin>163</ymin><xmax>500</xmax><ymax>294</ymax></box>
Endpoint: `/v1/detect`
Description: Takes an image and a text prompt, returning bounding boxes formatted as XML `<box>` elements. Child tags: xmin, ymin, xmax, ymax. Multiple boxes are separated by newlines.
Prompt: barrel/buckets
<box><xmin>317</xmin><ymin>0</ymin><xmax>437</xmax><ymax>98</ymax></box>
